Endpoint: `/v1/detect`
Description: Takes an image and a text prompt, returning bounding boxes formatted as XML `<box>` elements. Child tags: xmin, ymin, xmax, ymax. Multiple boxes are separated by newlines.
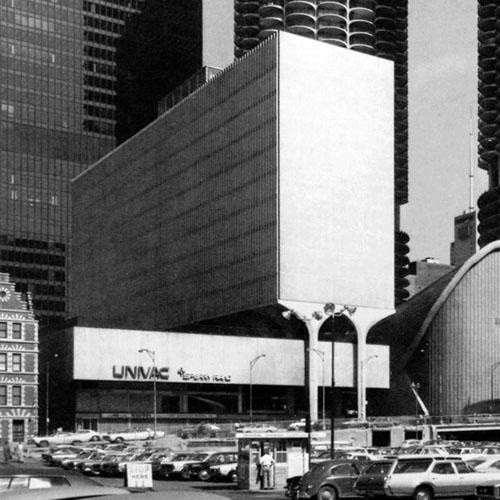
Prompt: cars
<box><xmin>343</xmin><ymin>406</ymin><xmax>358</xmax><ymax>417</ymax></box>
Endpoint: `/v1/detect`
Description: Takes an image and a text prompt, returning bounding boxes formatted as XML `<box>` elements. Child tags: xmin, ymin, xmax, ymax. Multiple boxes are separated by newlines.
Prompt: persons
<box><xmin>259</xmin><ymin>450</ymin><xmax>275</xmax><ymax>490</ymax></box>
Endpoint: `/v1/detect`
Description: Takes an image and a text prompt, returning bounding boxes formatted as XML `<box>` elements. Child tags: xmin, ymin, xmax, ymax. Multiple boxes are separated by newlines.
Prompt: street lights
<box><xmin>409</xmin><ymin>382</ymin><xmax>421</xmax><ymax>422</ymax></box>
<box><xmin>44</xmin><ymin>350</ymin><xmax>59</xmax><ymax>436</ymax></box>
<box><xmin>135</xmin><ymin>348</ymin><xmax>158</xmax><ymax>441</ymax></box>
<box><xmin>280</xmin><ymin>297</ymin><xmax>357</xmax><ymax>458</ymax></box>
<box><xmin>247</xmin><ymin>352</ymin><xmax>267</xmax><ymax>424</ymax></box>
<box><xmin>360</xmin><ymin>352</ymin><xmax>379</xmax><ymax>426</ymax></box>
